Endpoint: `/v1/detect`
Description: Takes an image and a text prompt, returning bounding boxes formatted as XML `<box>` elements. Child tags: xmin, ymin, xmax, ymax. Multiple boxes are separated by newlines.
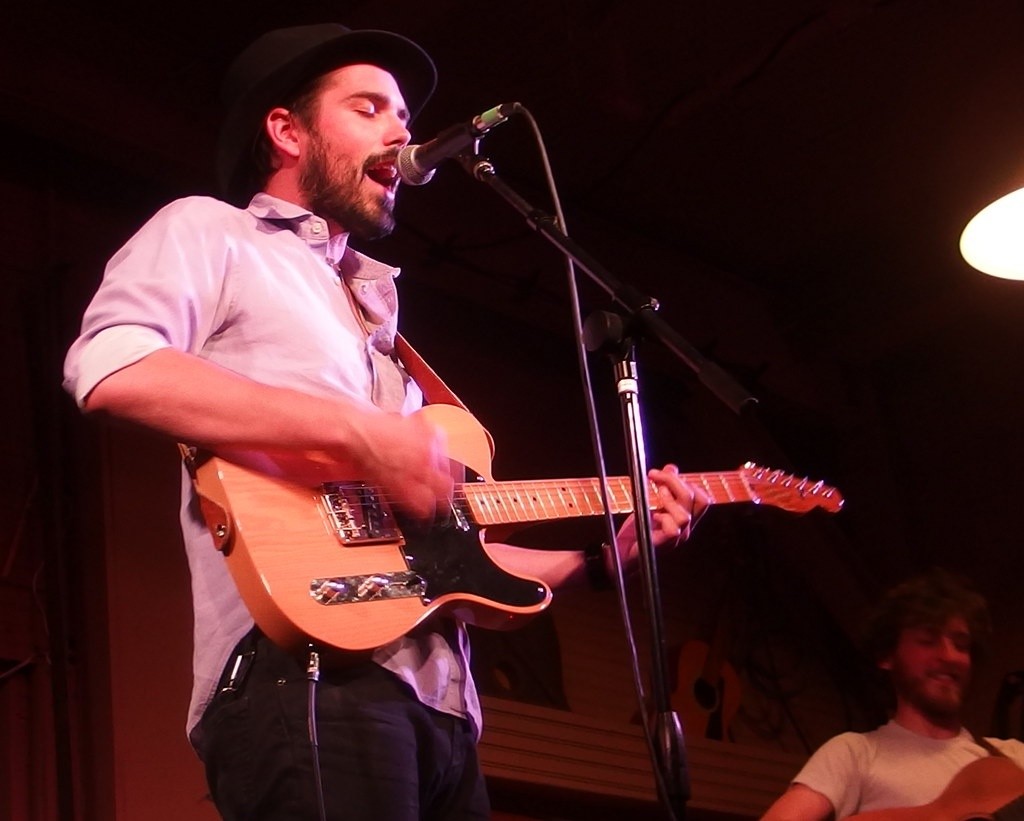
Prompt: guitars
<box><xmin>177</xmin><ymin>403</ymin><xmax>845</xmax><ymax>665</ymax></box>
<box><xmin>844</xmin><ymin>754</ymin><xmax>1024</xmax><ymax>821</ymax></box>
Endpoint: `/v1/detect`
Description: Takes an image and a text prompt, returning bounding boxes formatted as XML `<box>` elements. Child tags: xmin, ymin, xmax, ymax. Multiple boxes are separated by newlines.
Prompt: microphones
<box><xmin>395</xmin><ymin>102</ymin><xmax>521</xmax><ymax>186</ymax></box>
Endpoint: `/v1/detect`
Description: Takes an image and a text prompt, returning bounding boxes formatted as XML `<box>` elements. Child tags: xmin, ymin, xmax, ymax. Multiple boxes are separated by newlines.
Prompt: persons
<box><xmin>759</xmin><ymin>575</ymin><xmax>1024</xmax><ymax>821</ymax></box>
<box><xmin>63</xmin><ymin>22</ymin><xmax>710</xmax><ymax>821</ymax></box>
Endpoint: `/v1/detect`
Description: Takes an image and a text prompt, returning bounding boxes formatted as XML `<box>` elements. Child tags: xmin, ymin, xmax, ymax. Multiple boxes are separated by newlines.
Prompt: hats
<box><xmin>224</xmin><ymin>21</ymin><xmax>438</xmax><ymax>173</ymax></box>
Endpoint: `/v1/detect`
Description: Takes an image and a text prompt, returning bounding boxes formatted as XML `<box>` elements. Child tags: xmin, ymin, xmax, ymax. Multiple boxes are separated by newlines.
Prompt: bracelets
<box><xmin>584</xmin><ymin>542</ymin><xmax>614</xmax><ymax>591</ymax></box>
